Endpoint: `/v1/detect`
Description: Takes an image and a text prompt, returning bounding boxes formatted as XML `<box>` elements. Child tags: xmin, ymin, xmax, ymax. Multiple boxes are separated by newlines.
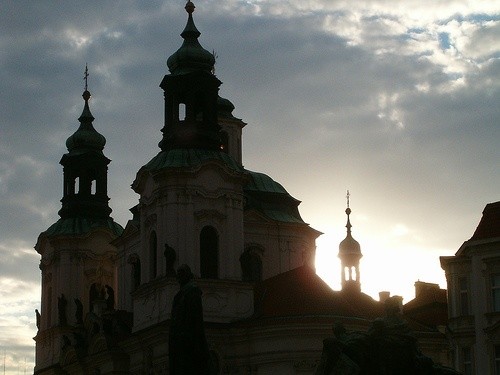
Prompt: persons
<box><xmin>240</xmin><ymin>245</ymin><xmax>258</xmax><ymax>281</ymax></box>
<box><xmin>166</xmin><ymin>263</ymin><xmax>205</xmax><ymax>371</ymax></box>
<box><xmin>159</xmin><ymin>241</ymin><xmax>178</xmax><ymax>277</ymax></box>
<box><xmin>56</xmin><ymin>292</ymin><xmax>69</xmax><ymax>327</ymax></box>
<box><xmin>314</xmin><ymin>322</ymin><xmax>356</xmax><ymax>375</ymax></box>
<box><xmin>335</xmin><ymin>296</ymin><xmax>428</xmax><ymax>375</ymax></box>
<box><xmin>33</xmin><ymin>308</ymin><xmax>41</xmax><ymax>335</ymax></box>
<box><xmin>103</xmin><ymin>284</ymin><xmax>115</xmax><ymax>310</ymax></box>
<box><xmin>72</xmin><ymin>294</ymin><xmax>86</xmax><ymax>325</ymax></box>
<box><xmin>87</xmin><ymin>282</ymin><xmax>99</xmax><ymax>312</ymax></box>
